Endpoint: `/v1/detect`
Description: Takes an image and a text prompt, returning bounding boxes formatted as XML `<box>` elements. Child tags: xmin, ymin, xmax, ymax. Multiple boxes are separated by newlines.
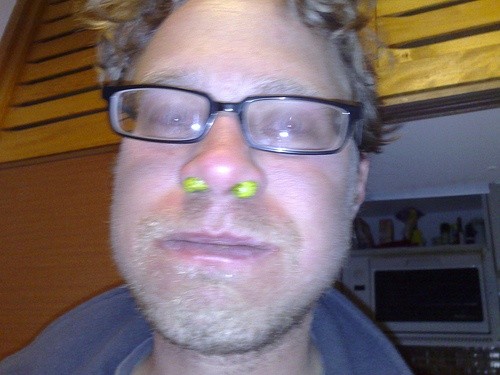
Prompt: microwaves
<box><xmin>339</xmin><ymin>252</ymin><xmax>491</xmax><ymax>334</ymax></box>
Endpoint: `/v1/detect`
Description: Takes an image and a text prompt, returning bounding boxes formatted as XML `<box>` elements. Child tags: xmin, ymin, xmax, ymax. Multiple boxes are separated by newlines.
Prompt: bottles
<box><xmin>355</xmin><ymin>214</ymin><xmax>375</xmax><ymax>249</ymax></box>
<box><xmin>403</xmin><ymin>210</ymin><xmax>421</xmax><ymax>245</ymax></box>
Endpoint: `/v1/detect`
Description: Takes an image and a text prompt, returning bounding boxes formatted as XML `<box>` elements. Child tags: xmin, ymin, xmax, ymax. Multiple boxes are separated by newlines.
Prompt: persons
<box><xmin>2</xmin><ymin>0</ymin><xmax>415</xmax><ymax>375</ymax></box>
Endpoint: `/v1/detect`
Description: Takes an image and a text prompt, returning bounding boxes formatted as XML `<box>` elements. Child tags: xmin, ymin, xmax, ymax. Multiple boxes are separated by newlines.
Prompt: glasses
<box><xmin>104</xmin><ymin>83</ymin><xmax>365</xmax><ymax>155</ymax></box>
